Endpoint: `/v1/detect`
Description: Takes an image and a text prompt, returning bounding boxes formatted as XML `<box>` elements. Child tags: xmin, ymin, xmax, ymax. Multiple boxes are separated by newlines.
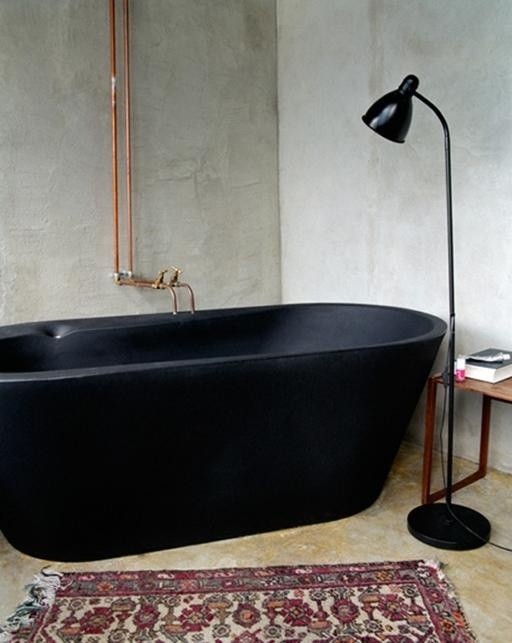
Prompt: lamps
<box><xmin>362</xmin><ymin>74</ymin><xmax>491</xmax><ymax>550</ymax></box>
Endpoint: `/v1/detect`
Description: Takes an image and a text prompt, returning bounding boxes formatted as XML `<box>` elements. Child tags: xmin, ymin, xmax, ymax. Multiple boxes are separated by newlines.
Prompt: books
<box><xmin>454</xmin><ymin>347</ymin><xmax>512</xmax><ymax>384</ymax></box>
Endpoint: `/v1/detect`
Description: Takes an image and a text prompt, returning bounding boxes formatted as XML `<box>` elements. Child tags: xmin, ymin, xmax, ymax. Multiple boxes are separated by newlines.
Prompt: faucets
<box><xmin>115</xmin><ymin>265</ymin><xmax>196</xmax><ymax>315</ymax></box>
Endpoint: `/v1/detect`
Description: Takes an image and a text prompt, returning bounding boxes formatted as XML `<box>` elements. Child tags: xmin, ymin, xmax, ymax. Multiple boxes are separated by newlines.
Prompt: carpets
<box><xmin>0</xmin><ymin>555</ymin><xmax>479</xmax><ymax>643</ymax></box>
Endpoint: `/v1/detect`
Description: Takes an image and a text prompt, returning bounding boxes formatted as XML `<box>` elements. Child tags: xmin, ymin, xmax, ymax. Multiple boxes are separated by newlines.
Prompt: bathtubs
<box><xmin>0</xmin><ymin>301</ymin><xmax>447</xmax><ymax>563</ymax></box>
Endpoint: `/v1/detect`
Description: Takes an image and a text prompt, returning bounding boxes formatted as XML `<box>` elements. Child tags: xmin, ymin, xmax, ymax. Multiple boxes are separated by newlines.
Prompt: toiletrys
<box><xmin>466</xmin><ymin>351</ymin><xmax>510</xmax><ymax>364</ymax></box>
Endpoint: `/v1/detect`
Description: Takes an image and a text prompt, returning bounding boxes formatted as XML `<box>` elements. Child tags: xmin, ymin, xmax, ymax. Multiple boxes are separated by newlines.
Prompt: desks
<box><xmin>422</xmin><ymin>372</ymin><xmax>512</xmax><ymax>504</ymax></box>
